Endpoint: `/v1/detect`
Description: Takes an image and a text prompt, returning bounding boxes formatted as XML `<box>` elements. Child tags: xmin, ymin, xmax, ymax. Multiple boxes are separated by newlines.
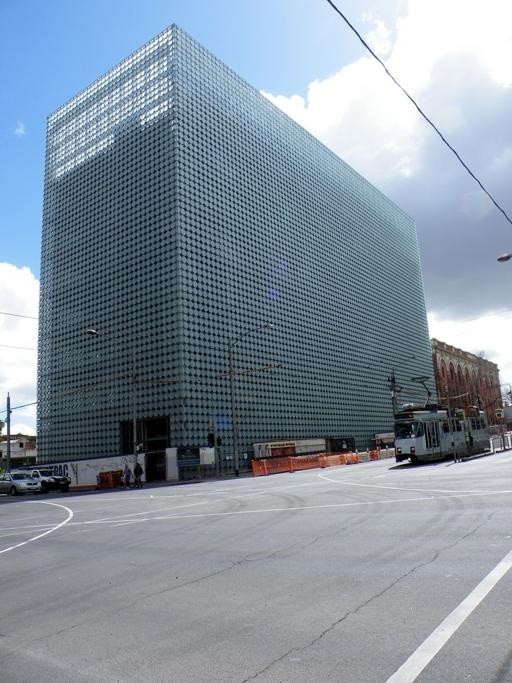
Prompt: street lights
<box><xmin>392</xmin><ymin>356</ymin><xmax>416</xmax><ymax>411</ymax></box>
<box><xmin>228</xmin><ymin>324</ymin><xmax>274</xmax><ymax>476</ymax></box>
<box><xmin>86</xmin><ymin>329</ymin><xmax>137</xmax><ymax>484</ymax></box>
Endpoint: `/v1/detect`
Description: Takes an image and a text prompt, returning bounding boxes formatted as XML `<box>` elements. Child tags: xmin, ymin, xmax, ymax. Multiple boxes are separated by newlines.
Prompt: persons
<box><xmin>136</xmin><ymin>463</ymin><xmax>147</xmax><ymax>489</ymax></box>
<box><xmin>468</xmin><ymin>432</ymin><xmax>475</xmax><ymax>451</ymax></box>
<box><xmin>123</xmin><ymin>465</ymin><xmax>134</xmax><ymax>491</ymax></box>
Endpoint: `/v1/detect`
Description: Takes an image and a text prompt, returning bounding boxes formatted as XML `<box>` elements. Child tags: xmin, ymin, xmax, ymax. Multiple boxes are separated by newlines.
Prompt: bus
<box><xmin>394</xmin><ymin>403</ymin><xmax>490</xmax><ymax>463</ymax></box>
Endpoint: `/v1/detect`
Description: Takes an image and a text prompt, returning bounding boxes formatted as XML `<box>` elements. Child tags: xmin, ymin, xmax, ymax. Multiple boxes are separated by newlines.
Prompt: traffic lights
<box><xmin>217</xmin><ymin>436</ymin><xmax>222</xmax><ymax>446</ymax></box>
<box><xmin>208</xmin><ymin>433</ymin><xmax>214</xmax><ymax>448</ymax></box>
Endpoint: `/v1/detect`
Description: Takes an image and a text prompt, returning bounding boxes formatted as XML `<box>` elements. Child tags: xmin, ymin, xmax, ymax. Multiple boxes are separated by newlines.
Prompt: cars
<box><xmin>0</xmin><ymin>469</ymin><xmax>71</xmax><ymax>496</ymax></box>
